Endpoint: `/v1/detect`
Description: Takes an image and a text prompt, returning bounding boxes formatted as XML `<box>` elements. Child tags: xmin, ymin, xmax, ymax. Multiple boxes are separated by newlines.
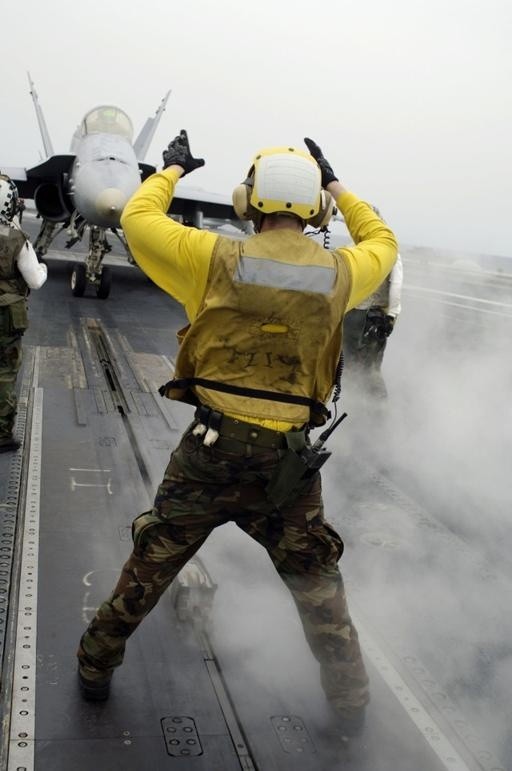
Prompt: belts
<box><xmin>218</xmin><ymin>415</ymin><xmax>284</xmax><ymax>450</ymax></box>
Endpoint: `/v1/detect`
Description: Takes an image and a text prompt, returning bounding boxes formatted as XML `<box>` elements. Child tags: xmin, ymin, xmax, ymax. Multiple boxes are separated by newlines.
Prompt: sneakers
<box><xmin>0</xmin><ymin>438</ymin><xmax>22</xmax><ymax>454</ymax></box>
<box><xmin>335</xmin><ymin>712</ymin><xmax>364</xmax><ymax>737</ymax></box>
<box><xmin>77</xmin><ymin>669</ymin><xmax>112</xmax><ymax>697</ymax></box>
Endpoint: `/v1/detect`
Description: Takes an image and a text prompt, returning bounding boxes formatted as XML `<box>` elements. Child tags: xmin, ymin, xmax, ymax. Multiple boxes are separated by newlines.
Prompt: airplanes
<box><xmin>1</xmin><ymin>72</ymin><xmax>240</xmax><ymax>297</ymax></box>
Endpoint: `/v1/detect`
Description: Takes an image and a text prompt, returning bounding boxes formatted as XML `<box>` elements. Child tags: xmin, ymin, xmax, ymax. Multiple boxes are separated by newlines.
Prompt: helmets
<box><xmin>245</xmin><ymin>145</ymin><xmax>322</xmax><ymax>221</ymax></box>
<box><xmin>0</xmin><ymin>174</ymin><xmax>19</xmax><ymax>221</ymax></box>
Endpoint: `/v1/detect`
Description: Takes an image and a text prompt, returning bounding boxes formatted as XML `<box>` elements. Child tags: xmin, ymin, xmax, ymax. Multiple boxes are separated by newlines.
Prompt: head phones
<box><xmin>1</xmin><ymin>174</ymin><xmax>25</xmax><ymax>215</ymax></box>
<box><xmin>232</xmin><ymin>183</ymin><xmax>338</xmax><ymax>228</ymax></box>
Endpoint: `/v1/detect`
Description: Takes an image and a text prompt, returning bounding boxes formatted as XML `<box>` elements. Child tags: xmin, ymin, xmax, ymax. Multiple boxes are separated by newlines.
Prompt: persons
<box><xmin>0</xmin><ymin>174</ymin><xmax>48</xmax><ymax>454</ymax></box>
<box><xmin>77</xmin><ymin>130</ymin><xmax>399</xmax><ymax>731</ymax></box>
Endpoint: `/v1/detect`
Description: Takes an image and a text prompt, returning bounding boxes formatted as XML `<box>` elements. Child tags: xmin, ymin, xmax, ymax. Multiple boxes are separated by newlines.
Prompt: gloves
<box><xmin>303</xmin><ymin>136</ymin><xmax>338</xmax><ymax>190</ymax></box>
<box><xmin>161</xmin><ymin>129</ymin><xmax>207</xmax><ymax>179</ymax></box>
